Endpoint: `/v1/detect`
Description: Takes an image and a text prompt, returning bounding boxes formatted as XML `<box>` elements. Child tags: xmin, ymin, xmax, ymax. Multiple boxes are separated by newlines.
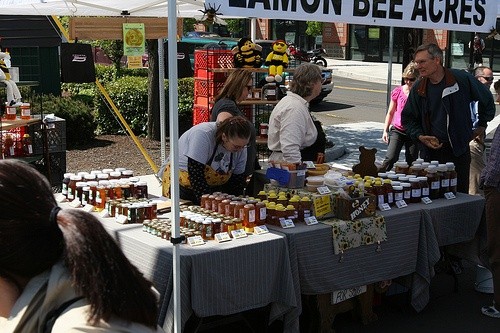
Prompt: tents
<box><xmin>0</xmin><ymin>1</ymin><xmax>243</xmax><ymax>333</ymax></box>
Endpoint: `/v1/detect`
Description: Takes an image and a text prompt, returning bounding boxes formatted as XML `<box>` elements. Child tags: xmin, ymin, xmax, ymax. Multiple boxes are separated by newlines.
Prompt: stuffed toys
<box><xmin>234</xmin><ymin>37</ymin><xmax>263</xmax><ymax>68</ymax></box>
<box><xmin>265</xmin><ymin>40</ymin><xmax>289</xmax><ymax>81</ymax></box>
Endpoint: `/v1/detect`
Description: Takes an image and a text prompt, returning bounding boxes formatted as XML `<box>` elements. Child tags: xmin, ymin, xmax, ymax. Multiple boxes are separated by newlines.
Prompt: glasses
<box><xmin>477</xmin><ymin>76</ymin><xmax>494</xmax><ymax>81</ymax></box>
<box><xmin>229</xmin><ymin>140</ymin><xmax>251</xmax><ymax>149</ymax></box>
<box><xmin>246</xmin><ymin>86</ymin><xmax>253</xmax><ymax>92</ymax></box>
<box><xmin>404</xmin><ymin>77</ymin><xmax>416</xmax><ymax>81</ymax></box>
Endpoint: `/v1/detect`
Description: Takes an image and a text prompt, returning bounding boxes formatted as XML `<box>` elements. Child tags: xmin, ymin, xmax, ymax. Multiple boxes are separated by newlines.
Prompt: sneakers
<box><xmin>482</xmin><ymin>305</ymin><xmax>500</xmax><ymax>318</ymax></box>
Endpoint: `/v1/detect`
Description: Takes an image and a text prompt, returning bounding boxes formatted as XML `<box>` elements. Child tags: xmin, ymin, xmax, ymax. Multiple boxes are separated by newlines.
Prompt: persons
<box><xmin>377</xmin><ymin>62</ymin><xmax>418</xmax><ymax>170</ymax></box>
<box><xmin>210</xmin><ymin>69</ymin><xmax>261</xmax><ymax>196</ymax></box>
<box><xmin>287</xmin><ymin>43</ymin><xmax>308</xmax><ymax>61</ymax></box>
<box><xmin>469</xmin><ymin>66</ymin><xmax>494</xmax><ymax>194</ymax></box>
<box><xmin>480</xmin><ymin>81</ymin><xmax>500</xmax><ymax>316</ymax></box>
<box><xmin>468</xmin><ymin>36</ymin><xmax>484</xmax><ymax>64</ymax></box>
<box><xmin>0</xmin><ymin>159</ymin><xmax>159</xmax><ymax>333</ymax></box>
<box><xmin>268</xmin><ymin>63</ymin><xmax>326</xmax><ymax>165</ymax></box>
<box><xmin>166</xmin><ymin>116</ymin><xmax>252</xmax><ymax>205</ymax></box>
<box><xmin>401</xmin><ymin>43</ymin><xmax>496</xmax><ymax>192</ymax></box>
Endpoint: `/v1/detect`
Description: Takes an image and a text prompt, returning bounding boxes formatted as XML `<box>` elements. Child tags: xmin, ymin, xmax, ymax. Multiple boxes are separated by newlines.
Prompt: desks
<box><xmin>51</xmin><ymin>182</ymin><xmax>303</xmax><ymax>333</ymax></box>
<box><xmin>271</xmin><ymin>191</ymin><xmax>485</xmax><ymax>333</ymax></box>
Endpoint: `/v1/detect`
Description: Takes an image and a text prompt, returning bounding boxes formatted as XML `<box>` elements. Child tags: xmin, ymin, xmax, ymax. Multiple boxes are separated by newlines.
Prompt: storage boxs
<box><xmin>17</xmin><ymin>119</ymin><xmax>66</xmax><ymax>195</ymax></box>
<box><xmin>333</xmin><ymin>192</ymin><xmax>376</xmax><ymax>219</ymax></box>
<box><xmin>193</xmin><ymin>48</ymin><xmax>253</xmax><ymax>128</ymax></box>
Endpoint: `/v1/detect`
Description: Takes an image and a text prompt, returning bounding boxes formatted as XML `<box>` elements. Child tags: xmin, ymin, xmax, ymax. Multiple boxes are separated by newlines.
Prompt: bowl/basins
<box><xmin>305</xmin><ymin>164</ymin><xmax>342</xmax><ymax>194</ymax></box>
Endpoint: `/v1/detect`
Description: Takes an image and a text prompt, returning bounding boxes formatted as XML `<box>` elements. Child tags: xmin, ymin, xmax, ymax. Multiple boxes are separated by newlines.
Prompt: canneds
<box><xmin>20</xmin><ymin>103</ymin><xmax>31</xmax><ymax>120</ymax></box>
<box><xmin>105</xmin><ymin>196</ymin><xmax>157</xmax><ymax>223</ymax></box>
<box><xmin>62</xmin><ymin>167</ymin><xmax>148</xmax><ymax>212</ymax></box>
<box><xmin>180</xmin><ymin>206</ymin><xmax>242</xmax><ymax>241</ymax></box>
<box><xmin>142</xmin><ymin>218</ymin><xmax>202</xmax><ymax>244</ymax></box>
<box><xmin>5</xmin><ymin>102</ymin><xmax>16</xmax><ymax>120</ymax></box>
<box><xmin>371</xmin><ymin>172</ymin><xmax>429</xmax><ymax>205</ymax></box>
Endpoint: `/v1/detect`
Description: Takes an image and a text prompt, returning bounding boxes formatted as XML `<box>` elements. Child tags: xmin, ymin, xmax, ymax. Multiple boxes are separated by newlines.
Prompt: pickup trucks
<box><xmin>180</xmin><ymin>35</ymin><xmax>334</xmax><ymax>106</ymax></box>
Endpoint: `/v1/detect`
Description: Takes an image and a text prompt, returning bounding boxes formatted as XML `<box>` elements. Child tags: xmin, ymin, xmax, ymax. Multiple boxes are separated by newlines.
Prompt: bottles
<box><xmin>143</xmin><ymin>191</ymin><xmax>313</xmax><ymax>246</ymax></box>
<box><xmin>267</xmin><ymin>83</ymin><xmax>276</xmax><ymax>101</ymax></box>
<box><xmin>253</xmin><ymin>89</ymin><xmax>259</xmax><ymax>98</ymax></box>
<box><xmin>264</xmin><ymin>158</ymin><xmax>307</xmax><ymax>186</ymax></box>
<box><xmin>247</xmin><ymin>89</ymin><xmax>252</xmax><ymax>98</ymax></box>
<box><xmin>5</xmin><ymin>101</ymin><xmax>31</xmax><ymax>119</ymax></box>
<box><xmin>3</xmin><ymin>48</ymin><xmax>11</xmax><ymax>68</ymax></box>
<box><xmin>62</xmin><ymin>167</ymin><xmax>157</xmax><ymax>224</ymax></box>
<box><xmin>260</xmin><ymin>123</ymin><xmax>269</xmax><ymax>138</ymax></box>
<box><xmin>346</xmin><ymin>159</ymin><xmax>458</xmax><ymax>207</ymax></box>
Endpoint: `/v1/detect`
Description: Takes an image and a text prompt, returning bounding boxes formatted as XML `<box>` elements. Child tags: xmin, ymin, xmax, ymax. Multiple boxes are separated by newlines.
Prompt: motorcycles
<box><xmin>304</xmin><ymin>47</ymin><xmax>328</xmax><ymax>67</ymax></box>
